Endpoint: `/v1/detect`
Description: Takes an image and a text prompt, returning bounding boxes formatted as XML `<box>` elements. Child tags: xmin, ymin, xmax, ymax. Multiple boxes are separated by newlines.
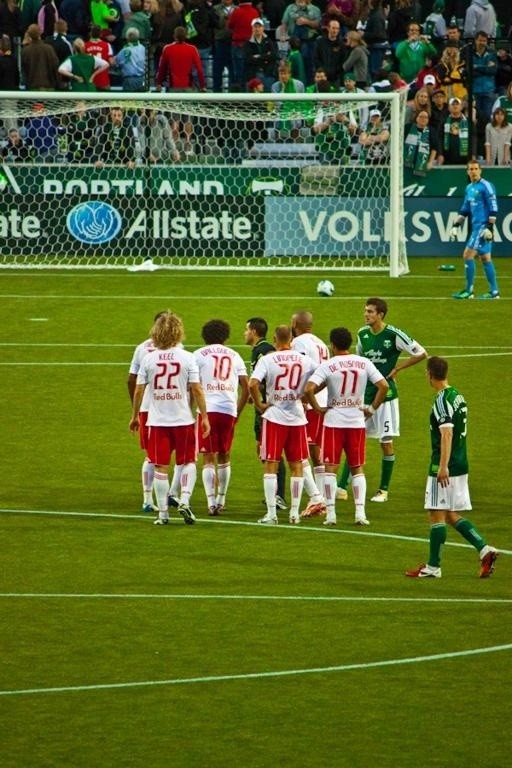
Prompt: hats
<box><xmin>448</xmin><ymin>97</ymin><xmax>461</xmax><ymax>105</ymax></box>
<box><xmin>369</xmin><ymin>109</ymin><xmax>381</xmax><ymax>117</ymax></box>
<box><xmin>247</xmin><ymin>78</ymin><xmax>263</xmax><ymax>90</ymax></box>
<box><xmin>430</xmin><ymin>89</ymin><xmax>445</xmax><ymax>97</ymax></box>
<box><xmin>432</xmin><ymin>0</ymin><xmax>446</xmax><ymax>14</ymax></box>
<box><xmin>424</xmin><ymin>74</ymin><xmax>436</xmax><ymax>85</ymax></box>
<box><xmin>251</xmin><ymin>17</ymin><xmax>264</xmax><ymax>27</ymax></box>
<box><xmin>343</xmin><ymin>71</ymin><xmax>356</xmax><ymax>80</ymax></box>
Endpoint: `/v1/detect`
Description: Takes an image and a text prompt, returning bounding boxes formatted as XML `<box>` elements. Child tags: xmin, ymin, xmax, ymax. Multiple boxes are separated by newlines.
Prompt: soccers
<box><xmin>317</xmin><ymin>280</ymin><xmax>335</xmax><ymax>297</ymax></box>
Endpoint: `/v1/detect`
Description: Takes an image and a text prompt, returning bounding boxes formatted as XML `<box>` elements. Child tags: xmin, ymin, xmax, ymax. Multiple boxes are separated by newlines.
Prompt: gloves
<box><xmin>450</xmin><ymin>222</ymin><xmax>461</xmax><ymax>238</ymax></box>
<box><xmin>480</xmin><ymin>222</ymin><xmax>493</xmax><ymax>241</ymax></box>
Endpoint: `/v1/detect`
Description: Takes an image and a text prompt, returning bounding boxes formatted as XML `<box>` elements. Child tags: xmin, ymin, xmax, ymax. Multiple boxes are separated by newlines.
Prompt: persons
<box><xmin>405</xmin><ymin>354</ymin><xmax>501</xmax><ymax>580</ymax></box>
<box><xmin>447</xmin><ymin>157</ymin><xmax>503</xmax><ymax>301</ymax></box>
<box><xmin>128</xmin><ymin>299</ymin><xmax>428</xmax><ymax>525</ymax></box>
<box><xmin>0</xmin><ymin>0</ymin><xmax>511</xmax><ymax>171</ymax></box>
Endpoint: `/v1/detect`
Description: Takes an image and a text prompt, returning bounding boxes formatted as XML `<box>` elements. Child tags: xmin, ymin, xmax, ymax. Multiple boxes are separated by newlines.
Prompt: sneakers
<box><xmin>405</xmin><ymin>561</ymin><xmax>442</xmax><ymax>578</ymax></box>
<box><xmin>208</xmin><ymin>504</ymin><xmax>224</xmax><ymax>516</ymax></box>
<box><xmin>370</xmin><ymin>489</ymin><xmax>388</xmax><ymax>502</ymax></box>
<box><xmin>142</xmin><ymin>503</ymin><xmax>159</xmax><ymax>512</ymax></box>
<box><xmin>451</xmin><ymin>290</ymin><xmax>474</xmax><ymax>299</ymax></box>
<box><xmin>480</xmin><ymin>290</ymin><xmax>500</xmax><ymax>299</ymax></box>
<box><xmin>177</xmin><ymin>503</ymin><xmax>196</xmax><ymax>525</ymax></box>
<box><xmin>152</xmin><ymin>517</ymin><xmax>168</xmax><ymax>525</ymax></box>
<box><xmin>479</xmin><ymin>546</ymin><xmax>500</xmax><ymax>578</ymax></box>
<box><xmin>257</xmin><ymin>493</ymin><xmax>370</xmax><ymax>525</ymax></box>
<box><xmin>335</xmin><ymin>487</ymin><xmax>348</xmax><ymax>500</ymax></box>
<box><xmin>167</xmin><ymin>493</ymin><xmax>180</xmax><ymax>508</ymax></box>
